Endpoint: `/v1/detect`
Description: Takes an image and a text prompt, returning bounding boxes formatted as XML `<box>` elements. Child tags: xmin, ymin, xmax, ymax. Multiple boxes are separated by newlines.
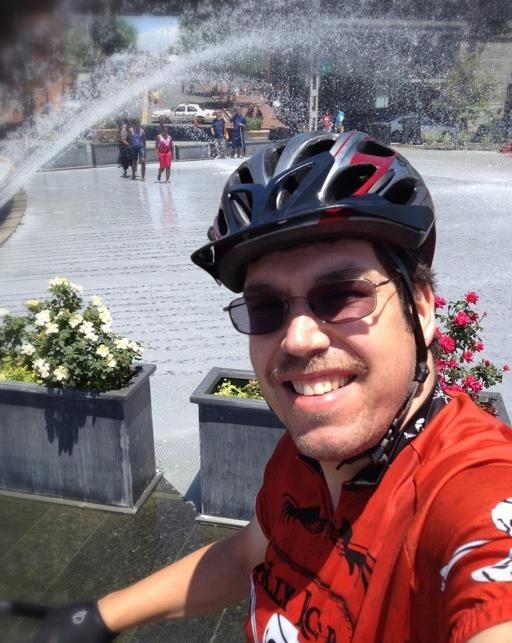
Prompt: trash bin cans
<box><xmin>369</xmin><ymin>122</ymin><xmax>391</xmax><ymax>147</ymax></box>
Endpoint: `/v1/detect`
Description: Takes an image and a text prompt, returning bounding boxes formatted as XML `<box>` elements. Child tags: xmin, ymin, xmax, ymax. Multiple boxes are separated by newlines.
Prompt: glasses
<box><xmin>223</xmin><ymin>274</ymin><xmax>401</xmax><ymax>337</ymax></box>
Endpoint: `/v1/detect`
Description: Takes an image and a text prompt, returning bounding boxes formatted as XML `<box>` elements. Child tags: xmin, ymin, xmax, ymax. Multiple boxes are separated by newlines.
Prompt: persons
<box><xmin>117</xmin><ymin>107</ymin><xmax>346</xmax><ymax>184</ymax></box>
<box><xmin>35</xmin><ymin>129</ymin><xmax>512</xmax><ymax>643</ymax></box>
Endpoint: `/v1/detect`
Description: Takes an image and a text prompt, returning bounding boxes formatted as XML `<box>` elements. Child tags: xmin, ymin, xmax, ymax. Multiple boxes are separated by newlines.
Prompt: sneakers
<box><xmin>213</xmin><ymin>154</ymin><xmax>245</xmax><ymax>158</ymax></box>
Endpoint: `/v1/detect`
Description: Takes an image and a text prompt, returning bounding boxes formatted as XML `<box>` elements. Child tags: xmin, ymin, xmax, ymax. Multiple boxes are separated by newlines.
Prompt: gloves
<box><xmin>31</xmin><ymin>599</ymin><xmax>120</xmax><ymax>643</ymax></box>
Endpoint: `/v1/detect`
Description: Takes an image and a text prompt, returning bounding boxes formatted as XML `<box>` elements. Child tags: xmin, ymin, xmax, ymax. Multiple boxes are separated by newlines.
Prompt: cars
<box><xmin>371</xmin><ymin>115</ymin><xmax>457</xmax><ymax>144</ymax></box>
<box><xmin>150</xmin><ymin>104</ymin><xmax>217</xmax><ymax>125</ymax></box>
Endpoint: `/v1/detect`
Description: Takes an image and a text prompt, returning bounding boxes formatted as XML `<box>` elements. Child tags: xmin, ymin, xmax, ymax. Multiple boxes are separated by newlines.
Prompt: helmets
<box><xmin>191</xmin><ymin>128</ymin><xmax>435</xmax><ymax>294</ymax></box>
